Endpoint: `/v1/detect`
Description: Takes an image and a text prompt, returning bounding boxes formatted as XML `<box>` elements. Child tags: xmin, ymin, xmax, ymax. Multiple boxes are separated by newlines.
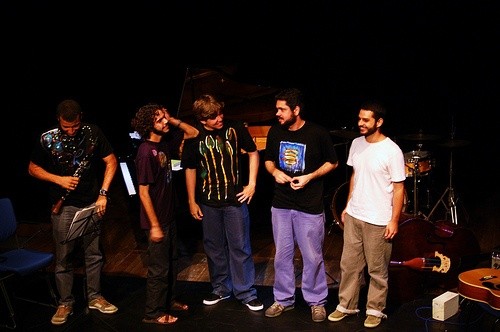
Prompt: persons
<box><xmin>28</xmin><ymin>100</ymin><xmax>118</xmax><ymax>324</ymax></box>
<box><xmin>327</xmin><ymin>106</ymin><xmax>407</xmax><ymax>328</ymax></box>
<box><xmin>265</xmin><ymin>88</ymin><xmax>339</xmax><ymax>322</ymax></box>
<box><xmin>131</xmin><ymin>103</ymin><xmax>199</xmax><ymax>325</ymax></box>
<box><xmin>180</xmin><ymin>94</ymin><xmax>264</xmax><ymax>311</ymax></box>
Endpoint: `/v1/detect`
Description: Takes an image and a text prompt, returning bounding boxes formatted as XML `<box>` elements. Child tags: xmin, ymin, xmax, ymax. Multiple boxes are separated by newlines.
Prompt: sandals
<box><xmin>161</xmin><ymin>300</ymin><xmax>190</xmax><ymax>311</ymax></box>
<box><xmin>142</xmin><ymin>313</ymin><xmax>178</xmax><ymax>325</ymax></box>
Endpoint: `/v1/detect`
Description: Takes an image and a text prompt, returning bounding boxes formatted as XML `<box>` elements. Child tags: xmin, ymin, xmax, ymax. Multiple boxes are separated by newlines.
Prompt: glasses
<box><xmin>202</xmin><ymin>108</ymin><xmax>224</xmax><ymax>120</ymax></box>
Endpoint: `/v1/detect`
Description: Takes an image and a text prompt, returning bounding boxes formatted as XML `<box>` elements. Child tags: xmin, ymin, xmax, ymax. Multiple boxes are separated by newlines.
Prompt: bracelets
<box><xmin>98</xmin><ymin>190</ymin><xmax>108</xmax><ymax>196</ymax></box>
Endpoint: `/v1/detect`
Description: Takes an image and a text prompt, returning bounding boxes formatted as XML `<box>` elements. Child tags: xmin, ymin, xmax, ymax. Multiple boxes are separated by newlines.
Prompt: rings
<box><xmin>73</xmin><ymin>184</ymin><xmax>75</xmax><ymax>188</ymax></box>
<box><xmin>192</xmin><ymin>214</ymin><xmax>194</xmax><ymax>217</ymax></box>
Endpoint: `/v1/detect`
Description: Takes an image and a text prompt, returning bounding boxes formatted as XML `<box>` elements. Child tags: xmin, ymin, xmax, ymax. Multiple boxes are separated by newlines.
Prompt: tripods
<box><xmin>398</xmin><ymin>121</ymin><xmax>458</xmax><ymax>235</ymax></box>
<box><xmin>59</xmin><ymin>209</ymin><xmax>119</xmax><ymax>332</ymax></box>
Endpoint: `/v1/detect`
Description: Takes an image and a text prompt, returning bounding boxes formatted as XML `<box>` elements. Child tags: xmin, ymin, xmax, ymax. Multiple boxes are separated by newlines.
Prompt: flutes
<box><xmin>50</xmin><ymin>141</ymin><xmax>97</xmax><ymax>217</ymax></box>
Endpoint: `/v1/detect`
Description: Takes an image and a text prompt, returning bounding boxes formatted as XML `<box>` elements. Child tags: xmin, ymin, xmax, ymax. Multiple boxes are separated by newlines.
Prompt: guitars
<box><xmin>457</xmin><ymin>268</ymin><xmax>500</xmax><ymax>311</ymax></box>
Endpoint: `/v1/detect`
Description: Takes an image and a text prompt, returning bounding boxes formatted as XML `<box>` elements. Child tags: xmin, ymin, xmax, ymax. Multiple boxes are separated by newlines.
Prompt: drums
<box><xmin>331</xmin><ymin>181</ymin><xmax>409</xmax><ymax>231</ymax></box>
<box><xmin>403</xmin><ymin>150</ymin><xmax>432</xmax><ymax>177</ymax></box>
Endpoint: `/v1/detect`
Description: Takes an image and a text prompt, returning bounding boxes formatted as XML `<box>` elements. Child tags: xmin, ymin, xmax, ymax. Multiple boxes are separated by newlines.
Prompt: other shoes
<box><xmin>363</xmin><ymin>315</ymin><xmax>381</xmax><ymax>328</ymax></box>
<box><xmin>51</xmin><ymin>304</ymin><xmax>73</xmax><ymax>324</ymax></box>
<box><xmin>264</xmin><ymin>301</ymin><xmax>294</xmax><ymax>317</ymax></box>
<box><xmin>89</xmin><ymin>296</ymin><xmax>117</xmax><ymax>313</ymax></box>
<box><xmin>328</xmin><ymin>310</ymin><xmax>356</xmax><ymax>322</ymax></box>
<box><xmin>245</xmin><ymin>299</ymin><xmax>264</xmax><ymax>310</ymax></box>
<box><xmin>311</xmin><ymin>305</ymin><xmax>326</xmax><ymax>322</ymax></box>
<box><xmin>202</xmin><ymin>294</ymin><xmax>231</xmax><ymax>305</ymax></box>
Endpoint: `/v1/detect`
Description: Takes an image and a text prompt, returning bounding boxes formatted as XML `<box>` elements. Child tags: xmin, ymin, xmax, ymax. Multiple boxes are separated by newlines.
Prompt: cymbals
<box><xmin>400</xmin><ymin>133</ymin><xmax>446</xmax><ymax>140</ymax></box>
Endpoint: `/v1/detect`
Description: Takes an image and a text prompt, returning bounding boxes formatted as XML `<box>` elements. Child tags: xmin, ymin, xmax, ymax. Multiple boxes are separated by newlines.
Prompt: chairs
<box><xmin>0</xmin><ymin>198</ymin><xmax>62</xmax><ymax>330</ymax></box>
